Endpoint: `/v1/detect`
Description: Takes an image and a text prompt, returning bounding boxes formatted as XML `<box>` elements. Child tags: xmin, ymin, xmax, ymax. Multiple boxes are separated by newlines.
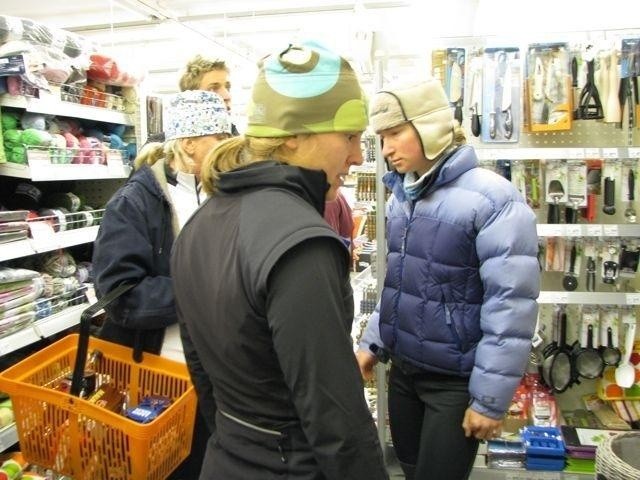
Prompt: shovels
<box><xmin>614</xmin><ymin>322</ymin><xmax>636</xmax><ymax>389</ymax></box>
<box><xmin>587</xmin><ymin>169</ymin><xmax>601</xmax><ymax>222</ymax></box>
<box><xmin>603</xmin><ymin>51</ymin><xmax>622</xmax><ymax>123</ymax></box>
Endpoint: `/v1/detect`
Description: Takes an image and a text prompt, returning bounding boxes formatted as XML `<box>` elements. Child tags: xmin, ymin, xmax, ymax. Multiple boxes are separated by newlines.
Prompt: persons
<box><xmin>145</xmin><ymin>55</ymin><xmax>240</xmax><ymax>142</ymax></box>
<box><xmin>168</xmin><ymin>42</ymin><xmax>389</xmax><ymax>480</ymax></box>
<box><xmin>323</xmin><ymin>191</ymin><xmax>354</xmax><ymax>272</ymax></box>
<box><xmin>355</xmin><ymin>71</ymin><xmax>542</xmax><ymax>480</ymax></box>
<box><xmin>90</xmin><ymin>89</ymin><xmax>233</xmax><ymax>480</ymax></box>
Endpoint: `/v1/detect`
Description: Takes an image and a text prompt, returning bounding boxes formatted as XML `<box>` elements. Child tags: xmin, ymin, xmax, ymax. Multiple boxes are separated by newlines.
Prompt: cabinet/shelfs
<box><xmin>0</xmin><ymin>66</ymin><xmax>150</xmax><ymax>453</ymax></box>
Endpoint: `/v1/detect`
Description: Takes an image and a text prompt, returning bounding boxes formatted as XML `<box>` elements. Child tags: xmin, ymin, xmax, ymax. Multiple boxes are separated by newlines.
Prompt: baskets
<box><xmin>0</xmin><ymin>270</ymin><xmax>196</xmax><ymax>480</ymax></box>
<box><xmin>592</xmin><ymin>429</ymin><xmax>640</xmax><ymax>479</ymax></box>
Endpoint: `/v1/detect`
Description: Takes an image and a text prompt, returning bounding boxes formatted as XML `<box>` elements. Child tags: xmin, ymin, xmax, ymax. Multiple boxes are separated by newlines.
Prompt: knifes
<box><xmin>450</xmin><ymin>51</ymin><xmax>555</xmax><ymax>139</ymax></box>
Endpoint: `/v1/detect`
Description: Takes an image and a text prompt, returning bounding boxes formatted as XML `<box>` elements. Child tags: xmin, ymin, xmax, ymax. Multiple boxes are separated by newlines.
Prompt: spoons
<box><xmin>624</xmin><ymin>170</ymin><xmax>637</xmax><ymax>223</ymax></box>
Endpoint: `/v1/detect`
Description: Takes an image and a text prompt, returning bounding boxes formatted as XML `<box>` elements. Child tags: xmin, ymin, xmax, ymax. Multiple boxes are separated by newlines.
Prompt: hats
<box><xmin>245</xmin><ymin>42</ymin><xmax>369</xmax><ymax>140</ymax></box>
<box><xmin>162</xmin><ymin>89</ymin><xmax>229</xmax><ymax>140</ymax></box>
<box><xmin>368</xmin><ymin>73</ymin><xmax>456</xmax><ymax>168</ymax></box>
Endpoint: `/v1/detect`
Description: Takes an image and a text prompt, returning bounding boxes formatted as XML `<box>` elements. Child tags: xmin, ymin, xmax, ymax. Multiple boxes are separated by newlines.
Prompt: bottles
<box><xmin>43</xmin><ymin>349</ymin><xmax>102</xmax><ymax>430</ymax></box>
